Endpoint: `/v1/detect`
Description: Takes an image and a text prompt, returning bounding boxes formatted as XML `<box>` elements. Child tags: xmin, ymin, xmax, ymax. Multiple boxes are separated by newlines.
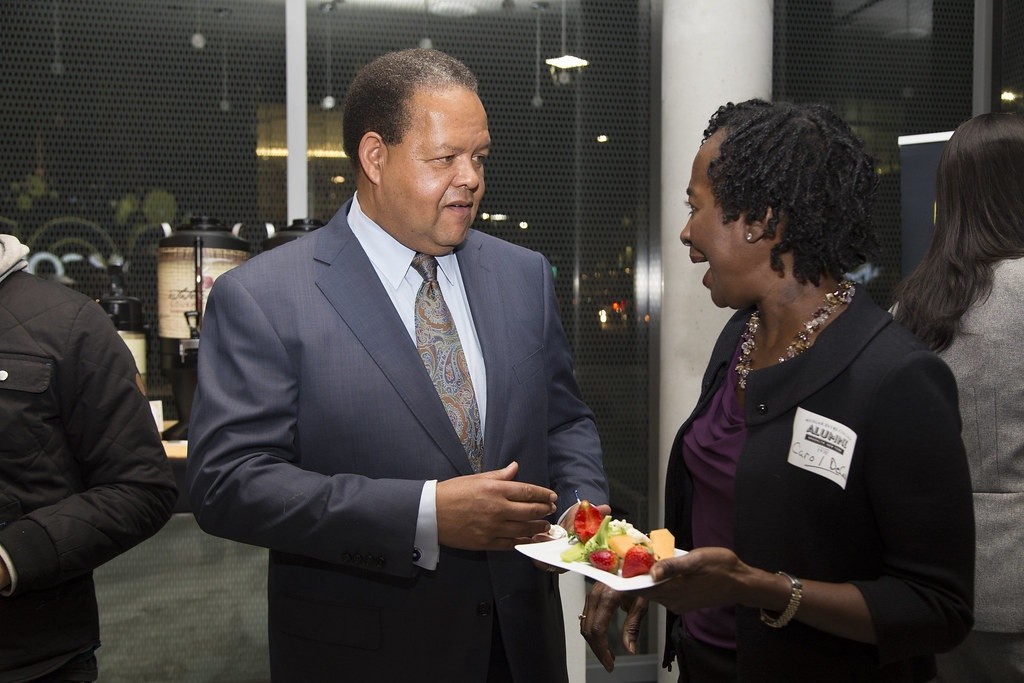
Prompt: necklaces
<box><xmin>734</xmin><ymin>278</ymin><xmax>856</xmax><ymax>388</ymax></box>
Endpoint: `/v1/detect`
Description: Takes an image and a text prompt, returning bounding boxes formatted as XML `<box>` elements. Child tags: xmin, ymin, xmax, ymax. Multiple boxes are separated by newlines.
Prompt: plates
<box><xmin>514</xmin><ymin>523</ymin><xmax>690</xmax><ymax>591</ymax></box>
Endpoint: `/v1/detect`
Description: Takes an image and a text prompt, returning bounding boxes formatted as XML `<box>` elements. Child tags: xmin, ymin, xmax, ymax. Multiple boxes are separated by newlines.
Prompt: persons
<box><xmin>187</xmin><ymin>47</ymin><xmax>609</xmax><ymax>682</ymax></box>
<box><xmin>577</xmin><ymin>98</ymin><xmax>978</xmax><ymax>682</ymax></box>
<box><xmin>884</xmin><ymin>110</ymin><xmax>1022</xmax><ymax>683</ymax></box>
<box><xmin>0</xmin><ymin>230</ymin><xmax>178</xmax><ymax>683</ymax></box>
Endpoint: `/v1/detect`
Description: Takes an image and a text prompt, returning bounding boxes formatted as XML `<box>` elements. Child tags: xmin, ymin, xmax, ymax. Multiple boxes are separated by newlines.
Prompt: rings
<box><xmin>577</xmin><ymin>613</ymin><xmax>588</xmax><ymax>620</ymax></box>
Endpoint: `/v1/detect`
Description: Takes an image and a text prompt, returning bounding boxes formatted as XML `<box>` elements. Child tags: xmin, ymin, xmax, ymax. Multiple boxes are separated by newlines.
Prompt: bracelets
<box><xmin>758</xmin><ymin>570</ymin><xmax>802</xmax><ymax>628</ymax></box>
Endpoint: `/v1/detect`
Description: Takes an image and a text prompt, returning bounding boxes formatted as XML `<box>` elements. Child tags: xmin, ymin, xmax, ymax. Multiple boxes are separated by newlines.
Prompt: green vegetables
<box><xmin>560</xmin><ymin>515</ymin><xmax>626</xmax><ymax>562</ymax></box>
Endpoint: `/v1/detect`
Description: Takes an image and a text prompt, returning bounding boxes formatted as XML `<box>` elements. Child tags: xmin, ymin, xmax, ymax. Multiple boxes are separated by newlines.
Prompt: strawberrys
<box><xmin>589</xmin><ymin>549</ymin><xmax>619</xmax><ymax>575</ymax></box>
<box><xmin>573</xmin><ymin>499</ymin><xmax>602</xmax><ymax>543</ymax></box>
<box><xmin>621</xmin><ymin>546</ymin><xmax>654</xmax><ymax>578</ymax></box>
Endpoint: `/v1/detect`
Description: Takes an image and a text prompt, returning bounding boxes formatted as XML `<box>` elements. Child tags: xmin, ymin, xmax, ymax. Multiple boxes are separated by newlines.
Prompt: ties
<box><xmin>409</xmin><ymin>250</ymin><xmax>484</xmax><ymax>473</ymax></box>
